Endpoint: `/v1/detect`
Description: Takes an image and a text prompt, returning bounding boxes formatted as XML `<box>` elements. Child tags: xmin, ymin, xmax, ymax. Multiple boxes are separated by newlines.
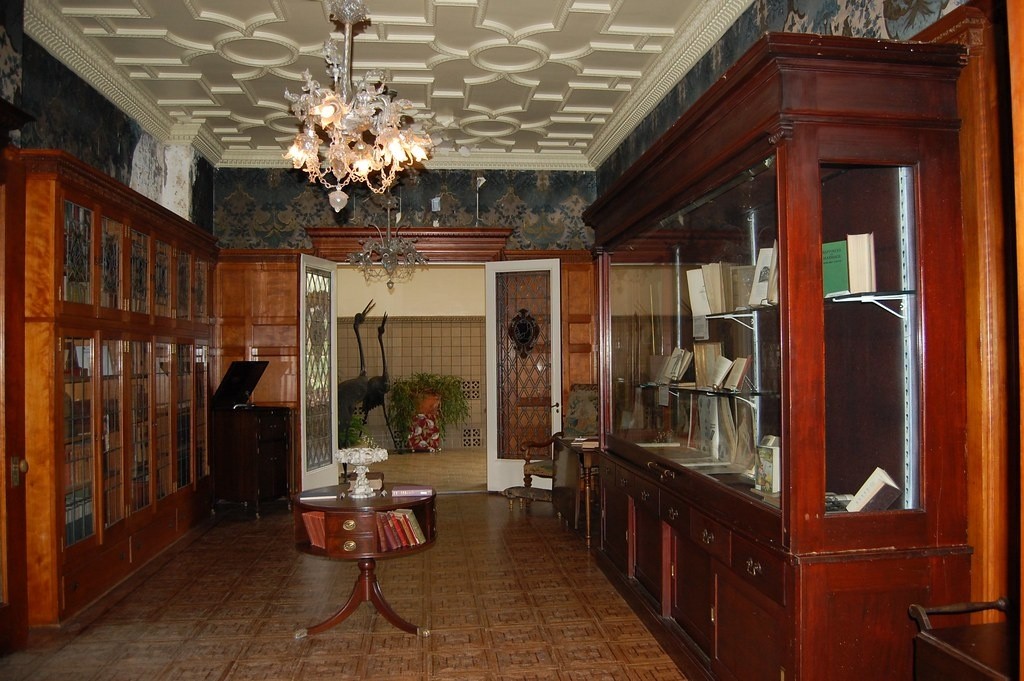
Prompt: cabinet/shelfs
<box><xmin>290</xmin><ymin>482</ymin><xmax>437</xmax><ymax>639</ymax></box>
<box><xmin>582</xmin><ymin>31</ymin><xmax>974</xmax><ymax>681</ymax></box>
<box><xmin>209</xmin><ymin>404</ymin><xmax>292</xmax><ymax>520</ymax></box>
<box><xmin>8</xmin><ymin>149</ymin><xmax>219</xmax><ymax>651</ymax></box>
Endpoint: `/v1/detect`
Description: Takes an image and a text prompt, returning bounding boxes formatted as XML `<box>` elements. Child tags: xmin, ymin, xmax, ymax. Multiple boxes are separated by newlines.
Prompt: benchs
<box><xmin>501</xmin><ymin>487</ymin><xmax>553</xmax><ymax>511</ymax></box>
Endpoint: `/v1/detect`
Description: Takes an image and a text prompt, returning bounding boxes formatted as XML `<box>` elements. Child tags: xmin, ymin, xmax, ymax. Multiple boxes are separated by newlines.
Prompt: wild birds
<box><xmin>338</xmin><ymin>300</ymin><xmax>399</xmax><ymax>454</ymax></box>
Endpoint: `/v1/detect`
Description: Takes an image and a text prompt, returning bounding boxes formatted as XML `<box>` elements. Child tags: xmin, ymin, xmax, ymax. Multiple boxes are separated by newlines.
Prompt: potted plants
<box><xmin>383</xmin><ymin>371</ymin><xmax>471</xmax><ymax>445</ymax></box>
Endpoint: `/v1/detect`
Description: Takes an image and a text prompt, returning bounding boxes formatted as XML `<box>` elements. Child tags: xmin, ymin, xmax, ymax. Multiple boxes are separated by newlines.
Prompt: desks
<box><xmin>553</xmin><ymin>437</ymin><xmax>601</xmax><ymax>533</ymax></box>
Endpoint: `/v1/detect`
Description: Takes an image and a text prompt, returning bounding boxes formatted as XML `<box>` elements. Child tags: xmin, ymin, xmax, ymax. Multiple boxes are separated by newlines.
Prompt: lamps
<box><xmin>281</xmin><ymin>0</ymin><xmax>432</xmax><ymax>292</ymax></box>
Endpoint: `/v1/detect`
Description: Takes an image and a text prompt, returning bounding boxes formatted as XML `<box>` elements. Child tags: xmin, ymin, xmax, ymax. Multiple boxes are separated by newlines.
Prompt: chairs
<box><xmin>520</xmin><ymin>384</ymin><xmax>600</xmax><ymax>487</ymax></box>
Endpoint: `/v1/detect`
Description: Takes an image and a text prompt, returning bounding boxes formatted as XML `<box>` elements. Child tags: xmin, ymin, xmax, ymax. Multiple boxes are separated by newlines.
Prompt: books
<box><xmin>663</xmin><ymin>340</ymin><xmax>752</xmax><ymax>393</ymax></box>
<box><xmin>391</xmin><ymin>484</ymin><xmax>433</xmax><ymax>498</ymax></box>
<box><xmin>302</xmin><ymin>510</ymin><xmax>327</xmax><ymax>551</ymax></box>
<box><xmin>747</xmin><ymin>238</ymin><xmax>778</xmax><ymax>308</ymax></box>
<box><xmin>612</xmin><ymin>378</ymin><xmax>670</xmax><ymax>430</ymax></box>
<box><xmin>300</xmin><ymin>485</ymin><xmax>340</xmax><ymax>500</ymax></box>
<box><xmin>376</xmin><ymin>508</ymin><xmax>426</xmax><ymax>552</ymax></box>
<box><xmin>826</xmin><ymin>466</ymin><xmax>903</xmax><ymax>512</ymax></box>
<box><xmin>821</xmin><ymin>238</ymin><xmax>850</xmax><ymax>300</ymax></box>
<box><xmin>848</xmin><ymin>232</ymin><xmax>877</xmax><ymax>295</ymax></box>
<box><xmin>677</xmin><ymin>389</ymin><xmax>781</xmax><ymax>497</ymax></box>
<box><xmin>686</xmin><ymin>260</ymin><xmax>755</xmax><ymax>315</ymax></box>
